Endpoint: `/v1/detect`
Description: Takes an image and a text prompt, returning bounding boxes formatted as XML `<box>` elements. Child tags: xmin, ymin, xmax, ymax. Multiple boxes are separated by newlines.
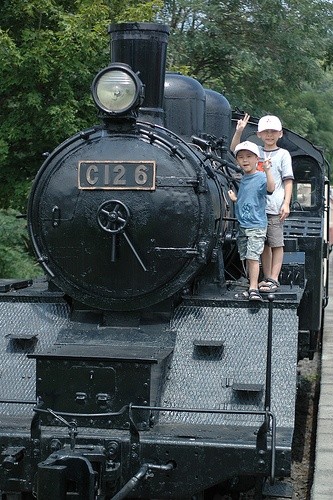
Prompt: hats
<box><xmin>233</xmin><ymin>141</ymin><xmax>260</xmax><ymax>158</ymax></box>
<box><xmin>258</xmin><ymin>115</ymin><xmax>282</xmax><ymax>133</ymax></box>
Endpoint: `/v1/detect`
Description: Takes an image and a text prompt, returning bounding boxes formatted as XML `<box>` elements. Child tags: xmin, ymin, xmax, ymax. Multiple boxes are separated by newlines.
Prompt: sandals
<box><xmin>234</xmin><ymin>290</ymin><xmax>250</xmax><ymax>300</ymax></box>
<box><xmin>249</xmin><ymin>289</ymin><xmax>262</xmax><ymax>301</ymax></box>
<box><xmin>259</xmin><ymin>278</ymin><xmax>280</xmax><ymax>292</ymax></box>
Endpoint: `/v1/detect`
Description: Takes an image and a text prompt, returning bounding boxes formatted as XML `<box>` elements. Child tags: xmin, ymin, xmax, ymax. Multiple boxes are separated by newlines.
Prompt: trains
<box><xmin>0</xmin><ymin>22</ymin><xmax>333</xmax><ymax>500</ymax></box>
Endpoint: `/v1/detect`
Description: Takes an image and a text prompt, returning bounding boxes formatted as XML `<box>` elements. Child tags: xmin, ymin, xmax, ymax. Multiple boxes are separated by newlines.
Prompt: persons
<box><xmin>228</xmin><ymin>141</ymin><xmax>275</xmax><ymax>302</ymax></box>
<box><xmin>230</xmin><ymin>113</ymin><xmax>294</xmax><ymax>293</ymax></box>
